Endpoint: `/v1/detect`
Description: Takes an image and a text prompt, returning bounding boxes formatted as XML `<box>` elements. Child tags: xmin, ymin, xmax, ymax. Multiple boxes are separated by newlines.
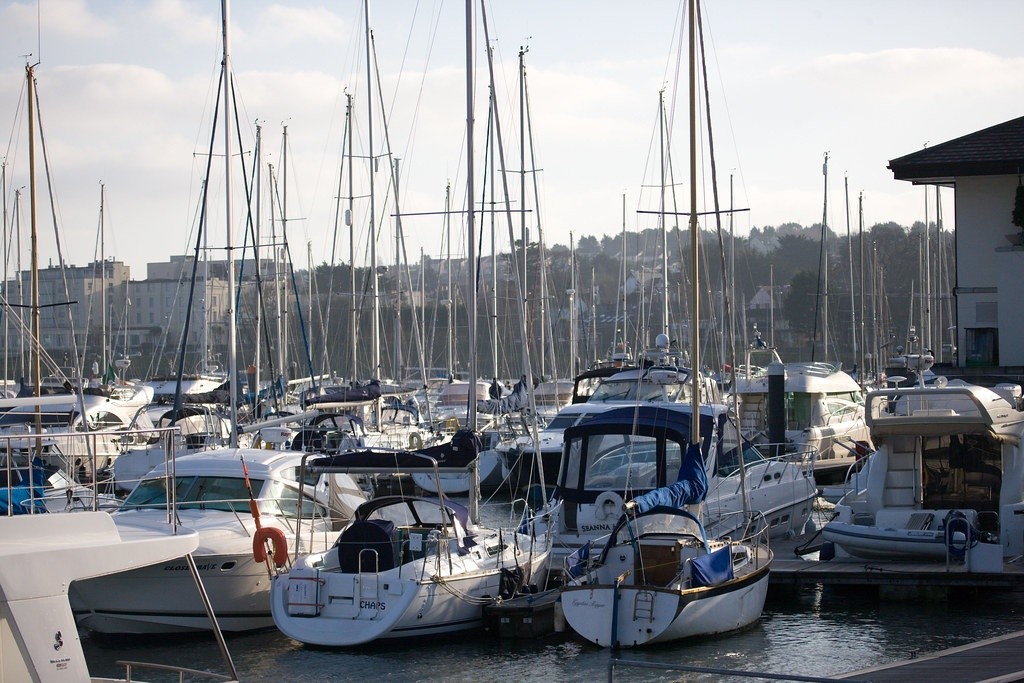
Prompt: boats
<box><xmin>0</xmin><ymin>0</ymin><xmax>550</xmax><ymax>649</ymax></box>
<box><xmin>497</xmin><ymin>0</ymin><xmax>1024</xmax><ymax>651</ymax></box>
